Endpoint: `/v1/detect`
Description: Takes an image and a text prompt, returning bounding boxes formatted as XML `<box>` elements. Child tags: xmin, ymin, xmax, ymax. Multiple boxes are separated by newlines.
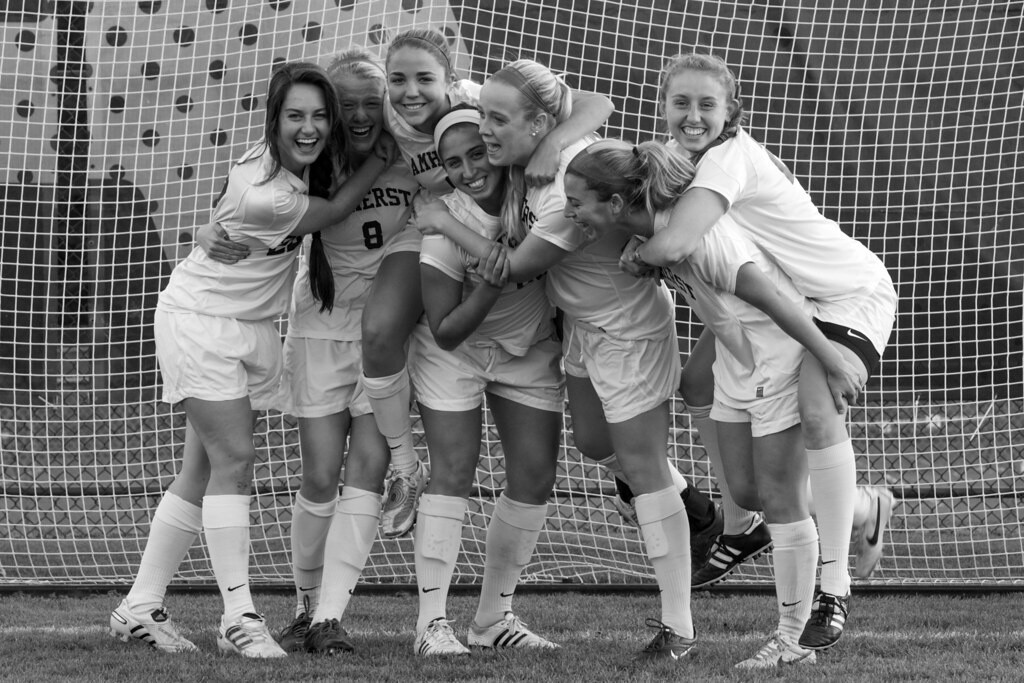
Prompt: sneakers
<box><xmin>216</xmin><ymin>612</ymin><xmax>289</xmax><ymax>658</ymax></box>
<box><xmin>636</xmin><ymin>626</ymin><xmax>700</xmax><ymax>663</ymax></box>
<box><xmin>855</xmin><ymin>485</ymin><xmax>893</xmax><ymax>578</ymax></box>
<box><xmin>613</xmin><ymin>493</ymin><xmax>642</xmax><ymax>533</ymax></box>
<box><xmin>690</xmin><ymin>500</ymin><xmax>724</xmax><ymax>576</ymax></box>
<box><xmin>278</xmin><ymin>611</ymin><xmax>313</xmax><ymax>652</ymax></box>
<box><xmin>466</xmin><ymin>610</ymin><xmax>563</xmax><ymax>651</ymax></box>
<box><xmin>381</xmin><ymin>457</ymin><xmax>428</xmax><ymax>539</ymax></box>
<box><xmin>413</xmin><ymin>616</ymin><xmax>471</xmax><ymax>657</ymax></box>
<box><xmin>303</xmin><ymin>618</ymin><xmax>355</xmax><ymax>654</ymax></box>
<box><xmin>109</xmin><ymin>599</ymin><xmax>201</xmax><ymax>653</ymax></box>
<box><xmin>798</xmin><ymin>591</ymin><xmax>853</xmax><ymax>649</ymax></box>
<box><xmin>734</xmin><ymin>630</ymin><xmax>817</xmax><ymax>669</ymax></box>
<box><xmin>690</xmin><ymin>513</ymin><xmax>773</xmax><ymax>588</ymax></box>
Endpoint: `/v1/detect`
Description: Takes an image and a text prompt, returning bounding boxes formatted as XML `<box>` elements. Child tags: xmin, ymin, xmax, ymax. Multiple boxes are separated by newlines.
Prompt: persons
<box><xmin>412</xmin><ymin>102</ymin><xmax>576</xmax><ymax>657</ymax></box>
<box><xmin>359</xmin><ymin>27</ymin><xmax>618</xmax><ymax>540</ymax></box>
<box><xmin>563</xmin><ymin>139</ymin><xmax>868</xmax><ymax>670</ymax></box>
<box><xmin>107</xmin><ymin>62</ymin><xmax>404</xmax><ymax>660</ymax></box>
<box><xmin>417</xmin><ymin>52</ymin><xmax>721</xmax><ymax>666</ymax></box>
<box><xmin>620</xmin><ymin>52</ymin><xmax>896</xmax><ymax>656</ymax></box>
<box><xmin>188</xmin><ymin>40</ymin><xmax>425</xmax><ymax>658</ymax></box>
